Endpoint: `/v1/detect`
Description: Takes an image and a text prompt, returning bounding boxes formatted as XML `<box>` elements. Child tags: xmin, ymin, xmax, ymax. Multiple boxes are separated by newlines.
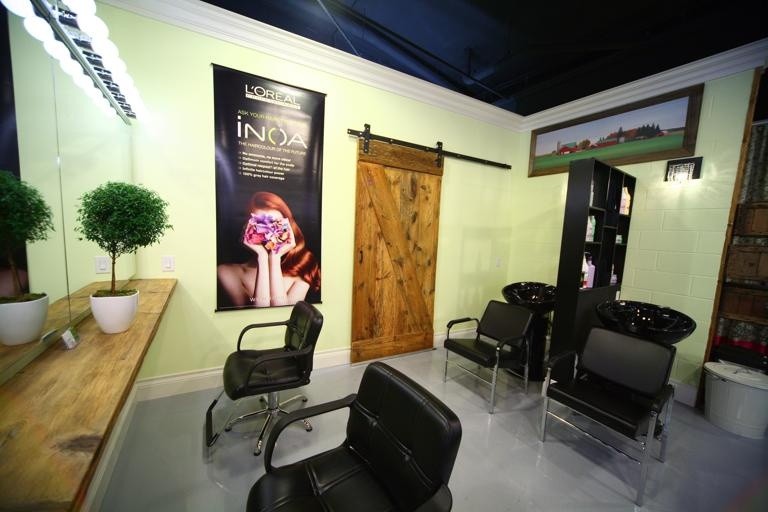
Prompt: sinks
<box><xmin>502</xmin><ymin>281</ymin><xmax>557</xmax><ymax>314</ymax></box>
<box><xmin>597</xmin><ymin>300</ymin><xmax>697</xmax><ymax>344</ymax></box>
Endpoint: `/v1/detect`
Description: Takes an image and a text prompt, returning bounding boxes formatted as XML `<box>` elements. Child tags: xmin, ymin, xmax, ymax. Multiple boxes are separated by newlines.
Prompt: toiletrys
<box><xmin>619</xmin><ymin>187</ymin><xmax>631</xmax><ymax>215</ymax></box>
<box><xmin>586</xmin><ymin>215</ymin><xmax>596</xmax><ymax>242</ymax></box>
<box><xmin>587</xmin><ymin>255</ymin><xmax>596</xmax><ymax>288</ymax></box>
<box><xmin>580</xmin><ymin>251</ymin><xmax>590</xmax><ymax>288</ymax></box>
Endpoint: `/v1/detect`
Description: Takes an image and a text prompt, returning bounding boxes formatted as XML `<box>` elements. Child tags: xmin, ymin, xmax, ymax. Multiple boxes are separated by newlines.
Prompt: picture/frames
<box><xmin>527</xmin><ymin>82</ymin><xmax>705</xmax><ymax>177</ymax></box>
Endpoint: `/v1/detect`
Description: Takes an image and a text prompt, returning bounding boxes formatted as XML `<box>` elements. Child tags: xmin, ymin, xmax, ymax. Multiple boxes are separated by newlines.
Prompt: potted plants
<box><xmin>73</xmin><ymin>181</ymin><xmax>175</xmax><ymax>333</ymax></box>
<box><xmin>0</xmin><ymin>168</ymin><xmax>54</xmax><ymax>347</ymax></box>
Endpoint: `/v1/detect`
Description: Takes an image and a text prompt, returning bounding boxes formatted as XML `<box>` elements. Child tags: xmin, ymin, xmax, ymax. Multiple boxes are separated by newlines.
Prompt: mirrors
<box><xmin>51</xmin><ymin>59</ymin><xmax>138</xmax><ymax>328</ymax></box>
<box><xmin>0</xmin><ymin>0</ymin><xmax>71</xmax><ymax>387</ymax></box>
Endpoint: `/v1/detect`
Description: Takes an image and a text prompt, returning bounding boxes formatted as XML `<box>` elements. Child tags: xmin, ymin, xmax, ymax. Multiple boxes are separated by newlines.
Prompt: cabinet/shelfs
<box><xmin>547</xmin><ymin>157</ymin><xmax>636</xmax><ymax>363</ymax></box>
<box><xmin>693</xmin><ymin>66</ymin><xmax>768</xmax><ymax>415</ymax></box>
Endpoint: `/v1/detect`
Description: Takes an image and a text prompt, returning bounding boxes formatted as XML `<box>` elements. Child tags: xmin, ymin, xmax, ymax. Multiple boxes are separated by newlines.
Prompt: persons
<box><xmin>216</xmin><ymin>190</ymin><xmax>321</xmax><ymax>308</ymax></box>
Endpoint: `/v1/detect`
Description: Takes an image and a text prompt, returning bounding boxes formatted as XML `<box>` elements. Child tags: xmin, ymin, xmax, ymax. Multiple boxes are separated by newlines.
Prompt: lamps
<box><xmin>665</xmin><ymin>157</ymin><xmax>702</xmax><ymax>183</ymax></box>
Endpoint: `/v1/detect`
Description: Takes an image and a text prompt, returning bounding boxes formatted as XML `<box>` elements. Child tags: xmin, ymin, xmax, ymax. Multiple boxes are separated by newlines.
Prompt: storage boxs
<box><xmin>703</xmin><ymin>360</ymin><xmax>768</xmax><ymax>440</ymax></box>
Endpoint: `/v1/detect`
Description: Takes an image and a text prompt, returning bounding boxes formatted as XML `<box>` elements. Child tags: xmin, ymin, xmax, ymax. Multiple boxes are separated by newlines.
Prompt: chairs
<box><xmin>244</xmin><ymin>360</ymin><xmax>462</xmax><ymax>512</ymax></box>
<box><xmin>204</xmin><ymin>301</ymin><xmax>325</xmax><ymax>455</ymax></box>
<box><xmin>445</xmin><ymin>299</ymin><xmax>533</xmax><ymax>414</ymax></box>
<box><xmin>538</xmin><ymin>325</ymin><xmax>676</xmax><ymax>507</ymax></box>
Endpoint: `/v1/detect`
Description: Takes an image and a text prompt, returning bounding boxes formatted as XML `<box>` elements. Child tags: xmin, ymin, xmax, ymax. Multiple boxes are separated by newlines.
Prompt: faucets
<box><xmin>545</xmin><ymin>284</ymin><xmax>556</xmax><ymax>293</ymax></box>
<box><xmin>615</xmin><ymin>299</ymin><xmax>626</xmax><ymax>307</ymax></box>
